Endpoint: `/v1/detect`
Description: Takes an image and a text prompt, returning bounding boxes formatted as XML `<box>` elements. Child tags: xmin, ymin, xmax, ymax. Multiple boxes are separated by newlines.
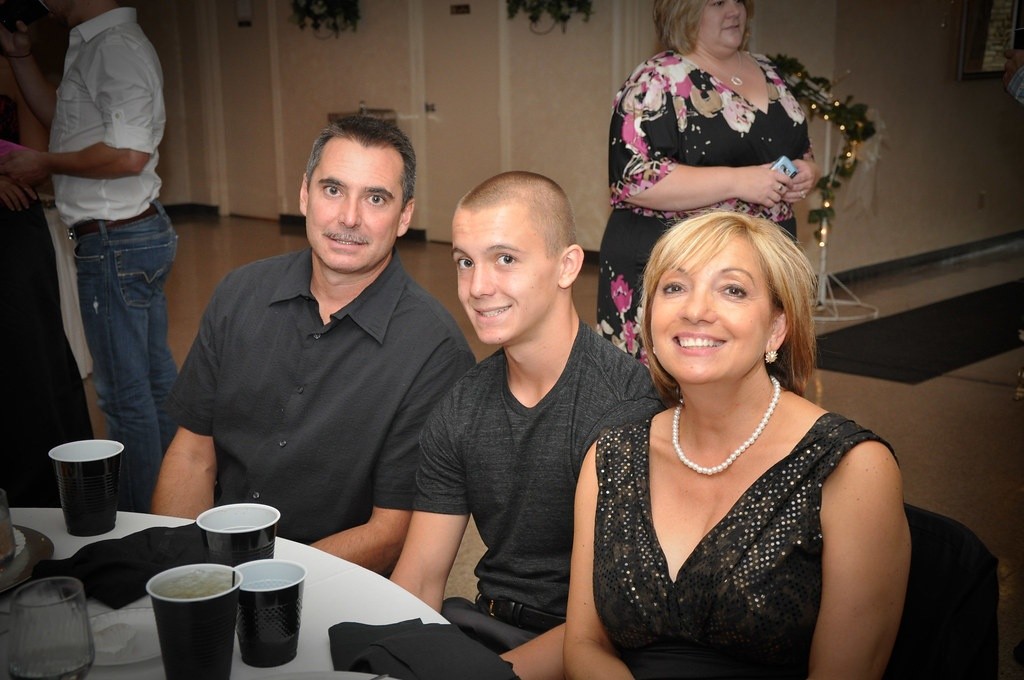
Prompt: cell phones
<box><xmin>770</xmin><ymin>155</ymin><xmax>799</xmax><ymax>177</ymax></box>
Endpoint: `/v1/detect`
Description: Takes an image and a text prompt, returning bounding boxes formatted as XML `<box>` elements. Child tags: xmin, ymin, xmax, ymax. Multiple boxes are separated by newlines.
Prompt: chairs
<box><xmin>883</xmin><ymin>503</ymin><xmax>1002</xmax><ymax>680</ymax></box>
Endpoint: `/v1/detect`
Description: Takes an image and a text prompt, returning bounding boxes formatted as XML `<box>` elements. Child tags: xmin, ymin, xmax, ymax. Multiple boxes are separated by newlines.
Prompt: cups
<box><xmin>146</xmin><ymin>562</ymin><xmax>244</xmax><ymax>680</ymax></box>
<box><xmin>48</xmin><ymin>439</ymin><xmax>125</xmax><ymax>537</ymax></box>
<box><xmin>232</xmin><ymin>559</ymin><xmax>310</xmax><ymax>669</ymax></box>
<box><xmin>196</xmin><ymin>502</ymin><xmax>282</xmax><ymax>567</ymax></box>
<box><xmin>0</xmin><ymin>489</ymin><xmax>17</xmax><ymax>565</ymax></box>
<box><xmin>8</xmin><ymin>576</ymin><xmax>95</xmax><ymax>680</ymax></box>
<box><xmin>0</xmin><ymin>0</ymin><xmax>50</xmax><ymax>34</ymax></box>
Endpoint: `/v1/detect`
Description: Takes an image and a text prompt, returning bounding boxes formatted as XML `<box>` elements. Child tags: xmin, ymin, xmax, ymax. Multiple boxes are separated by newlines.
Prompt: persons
<box><xmin>390</xmin><ymin>171</ymin><xmax>670</xmax><ymax>680</ymax></box>
<box><xmin>562</xmin><ymin>211</ymin><xmax>912</xmax><ymax>680</ymax></box>
<box><xmin>597</xmin><ymin>0</ymin><xmax>820</xmax><ymax>369</ymax></box>
<box><xmin>151</xmin><ymin>115</ymin><xmax>476</xmax><ymax>580</ymax></box>
<box><xmin>0</xmin><ymin>0</ymin><xmax>178</xmax><ymax>514</ymax></box>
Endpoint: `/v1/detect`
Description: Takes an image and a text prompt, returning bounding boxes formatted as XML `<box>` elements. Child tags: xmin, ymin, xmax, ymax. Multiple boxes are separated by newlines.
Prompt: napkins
<box><xmin>28</xmin><ymin>522</ymin><xmax>206</xmax><ymax>610</ymax></box>
<box><xmin>328</xmin><ymin>618</ymin><xmax>521</xmax><ymax>680</ymax></box>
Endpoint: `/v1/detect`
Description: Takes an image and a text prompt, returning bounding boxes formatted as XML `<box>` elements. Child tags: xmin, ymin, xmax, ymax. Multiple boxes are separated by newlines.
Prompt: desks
<box><xmin>0</xmin><ymin>507</ymin><xmax>450</xmax><ymax>680</ymax></box>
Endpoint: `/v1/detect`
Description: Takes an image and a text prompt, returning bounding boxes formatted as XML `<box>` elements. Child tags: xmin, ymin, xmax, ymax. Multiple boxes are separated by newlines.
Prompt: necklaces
<box><xmin>693</xmin><ymin>50</ymin><xmax>743</xmax><ymax>87</ymax></box>
<box><xmin>672</xmin><ymin>375</ymin><xmax>780</xmax><ymax>475</ymax></box>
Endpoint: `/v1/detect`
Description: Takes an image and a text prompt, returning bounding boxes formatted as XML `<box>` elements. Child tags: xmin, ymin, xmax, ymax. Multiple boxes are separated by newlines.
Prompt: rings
<box><xmin>800</xmin><ymin>191</ymin><xmax>807</xmax><ymax>199</ymax></box>
<box><xmin>777</xmin><ymin>186</ymin><xmax>782</xmax><ymax>192</ymax></box>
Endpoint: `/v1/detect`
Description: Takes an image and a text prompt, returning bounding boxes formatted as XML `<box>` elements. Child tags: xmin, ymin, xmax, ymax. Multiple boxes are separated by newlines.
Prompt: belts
<box><xmin>478</xmin><ymin>595</ymin><xmax>566</xmax><ymax>632</ymax></box>
<box><xmin>67</xmin><ymin>202</ymin><xmax>158</xmax><ymax>243</ymax></box>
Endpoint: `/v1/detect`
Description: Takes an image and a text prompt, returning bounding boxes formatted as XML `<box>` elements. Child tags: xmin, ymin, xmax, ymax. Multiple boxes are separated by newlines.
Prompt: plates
<box><xmin>86</xmin><ymin>606</ymin><xmax>161</xmax><ymax>667</ymax></box>
<box><xmin>0</xmin><ymin>524</ymin><xmax>55</xmax><ymax>594</ymax></box>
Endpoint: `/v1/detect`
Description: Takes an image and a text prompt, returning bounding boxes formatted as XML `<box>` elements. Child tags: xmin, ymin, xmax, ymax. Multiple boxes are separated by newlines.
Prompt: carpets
<box><xmin>813</xmin><ymin>278</ymin><xmax>1024</xmax><ymax>385</ymax></box>
